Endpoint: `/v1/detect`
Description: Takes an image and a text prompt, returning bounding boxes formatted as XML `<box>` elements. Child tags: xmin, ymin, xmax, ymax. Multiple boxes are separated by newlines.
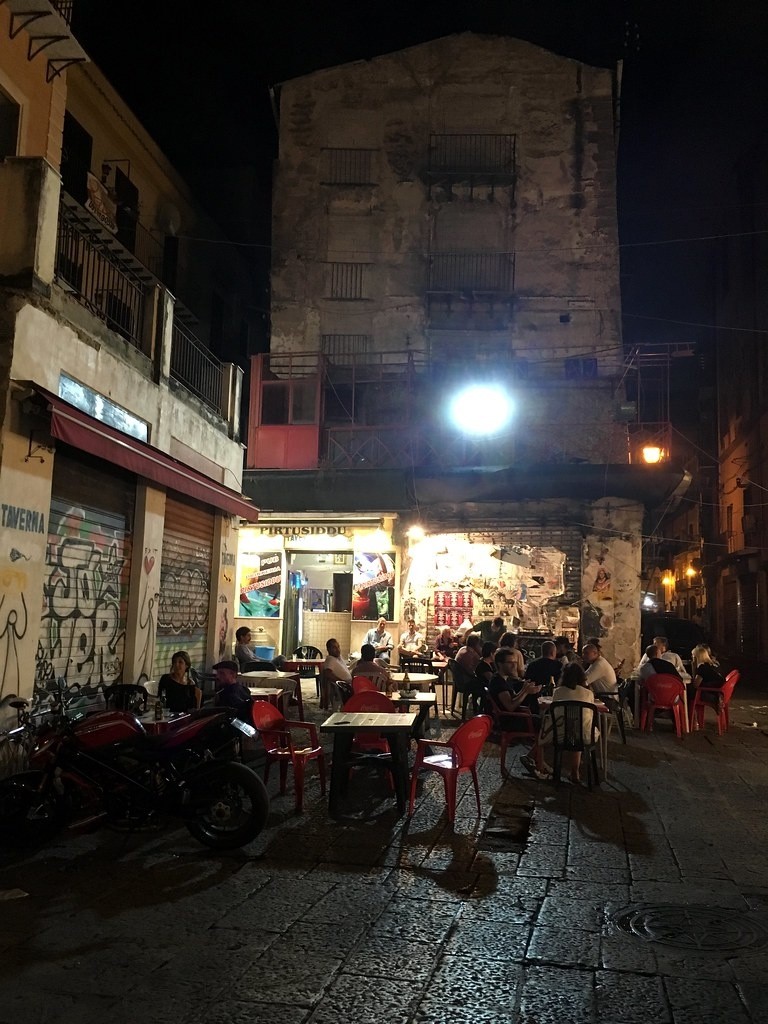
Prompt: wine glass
<box><xmin>302</xmin><ymin>647</ymin><xmax>307</xmax><ymax>660</ymax></box>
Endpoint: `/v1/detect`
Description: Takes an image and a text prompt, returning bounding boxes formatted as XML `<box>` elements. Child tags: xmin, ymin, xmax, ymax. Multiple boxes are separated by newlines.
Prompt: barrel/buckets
<box><xmin>255</xmin><ymin>646</ymin><xmax>275</xmax><ymax>661</ymax></box>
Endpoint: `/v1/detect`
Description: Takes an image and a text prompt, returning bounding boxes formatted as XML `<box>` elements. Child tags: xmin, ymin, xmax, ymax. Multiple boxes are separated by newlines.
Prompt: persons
<box><xmin>323</xmin><ymin>617</ymin><xmax>400</xmax><ymax>691</ymax></box>
<box><xmin>398</xmin><ymin>620</ymin><xmax>427</xmax><ymax>659</ymax></box>
<box><xmin>208</xmin><ymin>660</ymin><xmax>252</xmax><ymax>723</ymax></box>
<box><xmin>235</xmin><ymin>627</ymin><xmax>291</xmax><ymax>672</ymax></box>
<box><xmin>432</xmin><ymin>617</ymin><xmax>619</xmax><ymax>785</ymax></box>
<box><xmin>158</xmin><ymin>650</ymin><xmax>202</xmax><ymax>715</ymax></box>
<box><xmin>635</xmin><ymin>635</ymin><xmax>726</xmax><ymax>733</ymax></box>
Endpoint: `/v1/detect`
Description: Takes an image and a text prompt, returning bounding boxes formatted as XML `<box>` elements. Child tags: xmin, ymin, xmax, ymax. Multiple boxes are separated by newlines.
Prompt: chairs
<box><xmin>409</xmin><ymin>713</ymin><xmax>493</xmax><ymax>825</ymax></box>
<box><xmin>252</xmin><ymin>701</ymin><xmax>326</xmax><ymax>814</ymax></box>
<box><xmin>104</xmin><ymin>645</ymin><xmax>740</xmax><ymax>794</ymax></box>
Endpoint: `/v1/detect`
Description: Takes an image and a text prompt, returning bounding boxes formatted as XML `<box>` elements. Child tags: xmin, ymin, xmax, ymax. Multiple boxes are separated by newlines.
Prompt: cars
<box><xmin>641</xmin><ymin>616</ymin><xmax>704</xmax><ymax>672</ymax></box>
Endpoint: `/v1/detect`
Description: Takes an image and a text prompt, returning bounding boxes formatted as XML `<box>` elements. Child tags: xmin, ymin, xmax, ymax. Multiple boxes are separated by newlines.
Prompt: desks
<box><xmin>319</xmin><ymin>712</ymin><xmax>415</xmax><ymax>816</ymax></box>
<box><xmin>278</xmin><ymin>657</ymin><xmax>328</xmax><ymax>708</ymax></box>
<box><xmin>133</xmin><ymin>710</ymin><xmax>191</xmax><ymax>737</ymax></box>
<box><xmin>391</xmin><ymin>672</ymin><xmax>439</xmax><ymax>695</ymax></box>
<box><xmin>537</xmin><ymin>696</ymin><xmax>613</xmax><ymax>783</ymax></box>
<box><xmin>400</xmin><ymin>661</ymin><xmax>449</xmax><ymax>716</ymax></box>
<box><xmin>239</xmin><ymin>671</ymin><xmax>304</xmax><ymax>720</ymax></box>
<box><xmin>381</xmin><ymin>692</ymin><xmax>438</xmax><ymax>739</ymax></box>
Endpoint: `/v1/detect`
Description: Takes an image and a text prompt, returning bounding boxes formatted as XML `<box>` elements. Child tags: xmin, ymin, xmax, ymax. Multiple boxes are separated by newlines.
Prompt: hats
<box><xmin>212</xmin><ymin>661</ymin><xmax>239</xmax><ymax>673</ymax></box>
<box><xmin>587</xmin><ymin>637</ymin><xmax>602</xmax><ymax>648</ymax></box>
<box><xmin>554</xmin><ymin>636</ymin><xmax>575</xmax><ymax>649</ymax></box>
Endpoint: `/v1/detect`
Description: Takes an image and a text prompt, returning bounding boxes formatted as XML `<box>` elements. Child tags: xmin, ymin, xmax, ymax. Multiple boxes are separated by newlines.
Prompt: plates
<box><xmin>399</xmin><ymin>693</ymin><xmax>416</xmax><ymax>698</ymax></box>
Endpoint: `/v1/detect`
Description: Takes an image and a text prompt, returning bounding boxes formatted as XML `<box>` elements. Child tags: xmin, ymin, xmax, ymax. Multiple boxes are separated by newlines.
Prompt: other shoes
<box><xmin>520</xmin><ymin>754</ymin><xmax>549</xmax><ymax>780</ymax></box>
<box><xmin>567</xmin><ymin>772</ymin><xmax>582</xmax><ymax>784</ymax></box>
<box><xmin>289</xmin><ymin>697</ymin><xmax>298</xmax><ymax>706</ymax></box>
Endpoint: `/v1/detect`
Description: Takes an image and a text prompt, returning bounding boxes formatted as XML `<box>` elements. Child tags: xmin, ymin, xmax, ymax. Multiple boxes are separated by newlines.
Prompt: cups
<box><xmin>353</xmin><ymin>598</ymin><xmax>370</xmax><ymax>619</ymax></box>
<box><xmin>292</xmin><ymin>654</ymin><xmax>297</xmax><ymax>660</ymax></box>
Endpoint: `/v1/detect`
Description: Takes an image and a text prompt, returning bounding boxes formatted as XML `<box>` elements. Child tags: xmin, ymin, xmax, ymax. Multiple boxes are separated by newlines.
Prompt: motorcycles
<box><xmin>0</xmin><ymin>676</ymin><xmax>268</xmax><ymax>858</ymax></box>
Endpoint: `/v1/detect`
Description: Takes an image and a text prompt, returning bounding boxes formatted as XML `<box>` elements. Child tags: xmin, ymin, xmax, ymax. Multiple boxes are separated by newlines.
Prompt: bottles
<box><xmin>403</xmin><ymin>669</ymin><xmax>411</xmax><ymax>691</ymax></box>
<box><xmin>421</xmin><ymin>641</ymin><xmax>428</xmax><ymax>653</ymax></box>
<box><xmin>548</xmin><ymin>676</ymin><xmax>556</xmax><ymax>696</ymax></box>
<box><xmin>161</xmin><ymin>689</ymin><xmax>166</xmax><ymax>709</ymax></box>
<box><xmin>386</xmin><ymin>671</ymin><xmax>393</xmax><ymax>697</ymax></box>
<box><xmin>155</xmin><ymin>696</ymin><xmax>162</xmax><ymax>720</ymax></box>
